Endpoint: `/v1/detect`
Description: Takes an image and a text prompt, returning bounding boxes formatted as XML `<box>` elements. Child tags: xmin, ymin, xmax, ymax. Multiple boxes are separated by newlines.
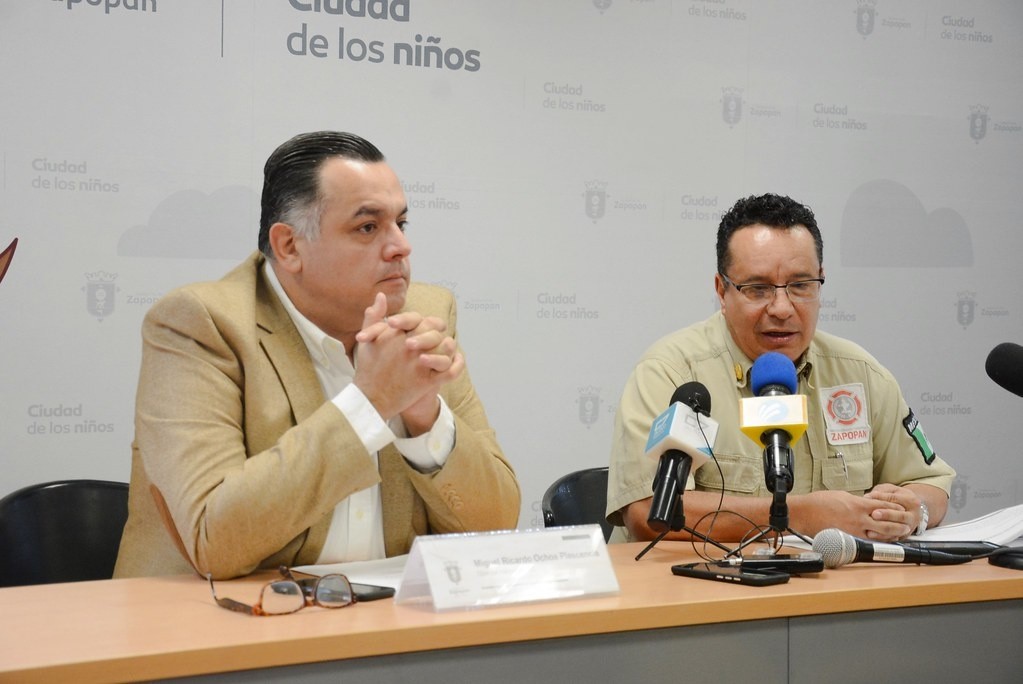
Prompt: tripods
<box><xmin>724</xmin><ymin>503</ymin><xmax>812</xmax><ymax>560</ymax></box>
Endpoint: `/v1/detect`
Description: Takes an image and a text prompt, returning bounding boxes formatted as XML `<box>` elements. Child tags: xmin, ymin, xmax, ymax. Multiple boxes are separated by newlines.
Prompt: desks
<box><xmin>0</xmin><ymin>542</ymin><xmax>1022</xmax><ymax>684</ymax></box>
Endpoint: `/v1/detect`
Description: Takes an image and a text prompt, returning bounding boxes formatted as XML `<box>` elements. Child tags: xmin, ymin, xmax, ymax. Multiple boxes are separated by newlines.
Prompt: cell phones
<box><xmin>743</xmin><ymin>552</ymin><xmax>823</xmax><ymax>573</ymax></box>
<box><xmin>670</xmin><ymin>560</ymin><xmax>790</xmax><ymax>587</ymax></box>
<box><xmin>906</xmin><ymin>541</ymin><xmax>1005</xmax><ymax>554</ymax></box>
<box><xmin>271</xmin><ymin>578</ymin><xmax>395</xmax><ymax>601</ymax></box>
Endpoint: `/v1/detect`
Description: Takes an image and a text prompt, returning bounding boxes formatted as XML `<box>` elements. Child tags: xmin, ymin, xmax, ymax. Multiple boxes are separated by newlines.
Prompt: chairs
<box><xmin>0</xmin><ymin>481</ymin><xmax>129</xmax><ymax>586</ymax></box>
<box><xmin>541</xmin><ymin>465</ymin><xmax>617</xmax><ymax>545</ymax></box>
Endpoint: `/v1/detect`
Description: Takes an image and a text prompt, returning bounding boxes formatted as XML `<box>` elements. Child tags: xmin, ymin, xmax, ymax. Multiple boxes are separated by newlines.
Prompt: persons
<box><xmin>111</xmin><ymin>131</ymin><xmax>521</xmax><ymax>578</ymax></box>
<box><xmin>602</xmin><ymin>193</ymin><xmax>959</xmax><ymax>545</ymax></box>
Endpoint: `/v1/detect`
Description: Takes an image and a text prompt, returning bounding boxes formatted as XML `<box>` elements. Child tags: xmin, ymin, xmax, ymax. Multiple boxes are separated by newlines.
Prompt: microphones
<box><xmin>634</xmin><ymin>381</ymin><xmax>719</xmax><ymax>531</ymax></box>
<box><xmin>812</xmin><ymin>527</ymin><xmax>971</xmax><ymax>567</ymax></box>
<box><xmin>985</xmin><ymin>343</ymin><xmax>1023</xmax><ymax>397</ymax></box>
<box><xmin>740</xmin><ymin>352</ymin><xmax>813</xmax><ymax>497</ymax></box>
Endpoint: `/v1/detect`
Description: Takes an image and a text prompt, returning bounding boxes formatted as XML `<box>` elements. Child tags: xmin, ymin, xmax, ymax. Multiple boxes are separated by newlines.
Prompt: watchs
<box><xmin>914</xmin><ymin>495</ymin><xmax>929</xmax><ymax>536</ymax></box>
